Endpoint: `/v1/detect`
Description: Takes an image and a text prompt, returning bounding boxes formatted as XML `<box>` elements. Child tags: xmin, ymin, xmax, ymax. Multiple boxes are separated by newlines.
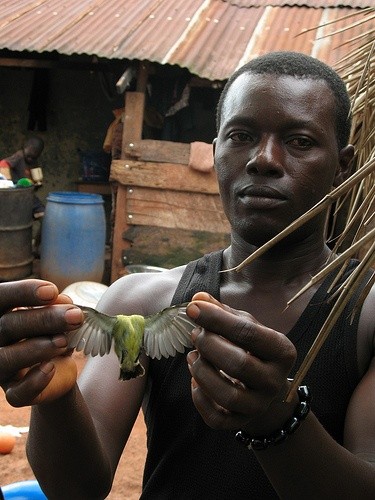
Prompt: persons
<box><xmin>0</xmin><ymin>51</ymin><xmax>375</xmax><ymax>500</ymax></box>
<box><xmin>0</xmin><ymin>138</ymin><xmax>45</xmax><ymax>259</ymax></box>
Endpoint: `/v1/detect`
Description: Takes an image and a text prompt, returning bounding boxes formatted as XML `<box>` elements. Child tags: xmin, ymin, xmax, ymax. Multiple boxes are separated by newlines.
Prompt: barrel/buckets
<box><xmin>39</xmin><ymin>191</ymin><xmax>106</xmax><ymax>295</ymax></box>
<box><xmin>0</xmin><ymin>186</ymin><xmax>34</xmax><ymax>281</ymax></box>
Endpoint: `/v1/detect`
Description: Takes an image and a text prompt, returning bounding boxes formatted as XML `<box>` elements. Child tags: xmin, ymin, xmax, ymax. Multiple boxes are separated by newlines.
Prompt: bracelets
<box><xmin>233</xmin><ymin>377</ymin><xmax>312</xmax><ymax>451</ymax></box>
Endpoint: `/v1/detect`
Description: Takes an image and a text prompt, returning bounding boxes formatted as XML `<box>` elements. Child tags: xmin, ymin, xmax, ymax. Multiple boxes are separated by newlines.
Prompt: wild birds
<box><xmin>65</xmin><ymin>302</ymin><xmax>199</xmax><ymax>385</ymax></box>
